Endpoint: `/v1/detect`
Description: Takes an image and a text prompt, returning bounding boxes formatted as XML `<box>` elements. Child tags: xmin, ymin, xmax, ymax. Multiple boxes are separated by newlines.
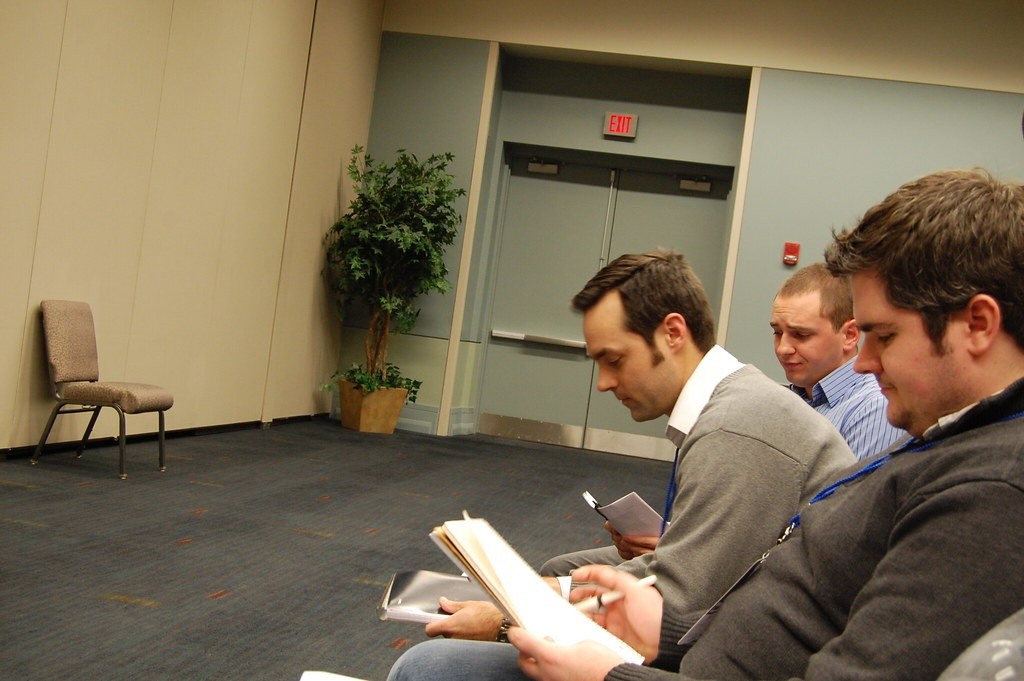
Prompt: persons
<box><xmin>387</xmin><ymin>167</ymin><xmax>1023</xmax><ymax>680</ymax></box>
<box><xmin>425</xmin><ymin>245</ymin><xmax>862</xmax><ymax>641</ymax></box>
<box><xmin>539</xmin><ymin>261</ymin><xmax>908</xmax><ymax>577</ymax></box>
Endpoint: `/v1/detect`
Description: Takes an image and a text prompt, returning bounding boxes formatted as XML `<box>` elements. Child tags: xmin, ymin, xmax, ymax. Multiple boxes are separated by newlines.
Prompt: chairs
<box><xmin>31</xmin><ymin>300</ymin><xmax>174</xmax><ymax>481</ymax></box>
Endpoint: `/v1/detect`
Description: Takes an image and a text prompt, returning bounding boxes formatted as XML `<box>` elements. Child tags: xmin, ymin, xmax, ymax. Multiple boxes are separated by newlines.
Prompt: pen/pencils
<box><xmin>572</xmin><ymin>574</ymin><xmax>658</xmax><ymax>616</ymax></box>
<box><xmin>583</xmin><ymin>491</ymin><xmax>609</xmax><ymax>520</ymax></box>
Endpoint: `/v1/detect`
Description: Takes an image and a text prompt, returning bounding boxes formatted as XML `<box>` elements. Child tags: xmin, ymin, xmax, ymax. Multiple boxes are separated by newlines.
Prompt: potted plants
<box><xmin>319</xmin><ymin>145</ymin><xmax>468</xmax><ymax>435</ymax></box>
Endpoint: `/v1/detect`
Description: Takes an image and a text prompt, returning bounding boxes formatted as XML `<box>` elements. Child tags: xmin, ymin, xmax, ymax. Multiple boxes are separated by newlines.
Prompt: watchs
<box><xmin>495</xmin><ymin>616</ymin><xmax>514</xmax><ymax>644</ymax></box>
